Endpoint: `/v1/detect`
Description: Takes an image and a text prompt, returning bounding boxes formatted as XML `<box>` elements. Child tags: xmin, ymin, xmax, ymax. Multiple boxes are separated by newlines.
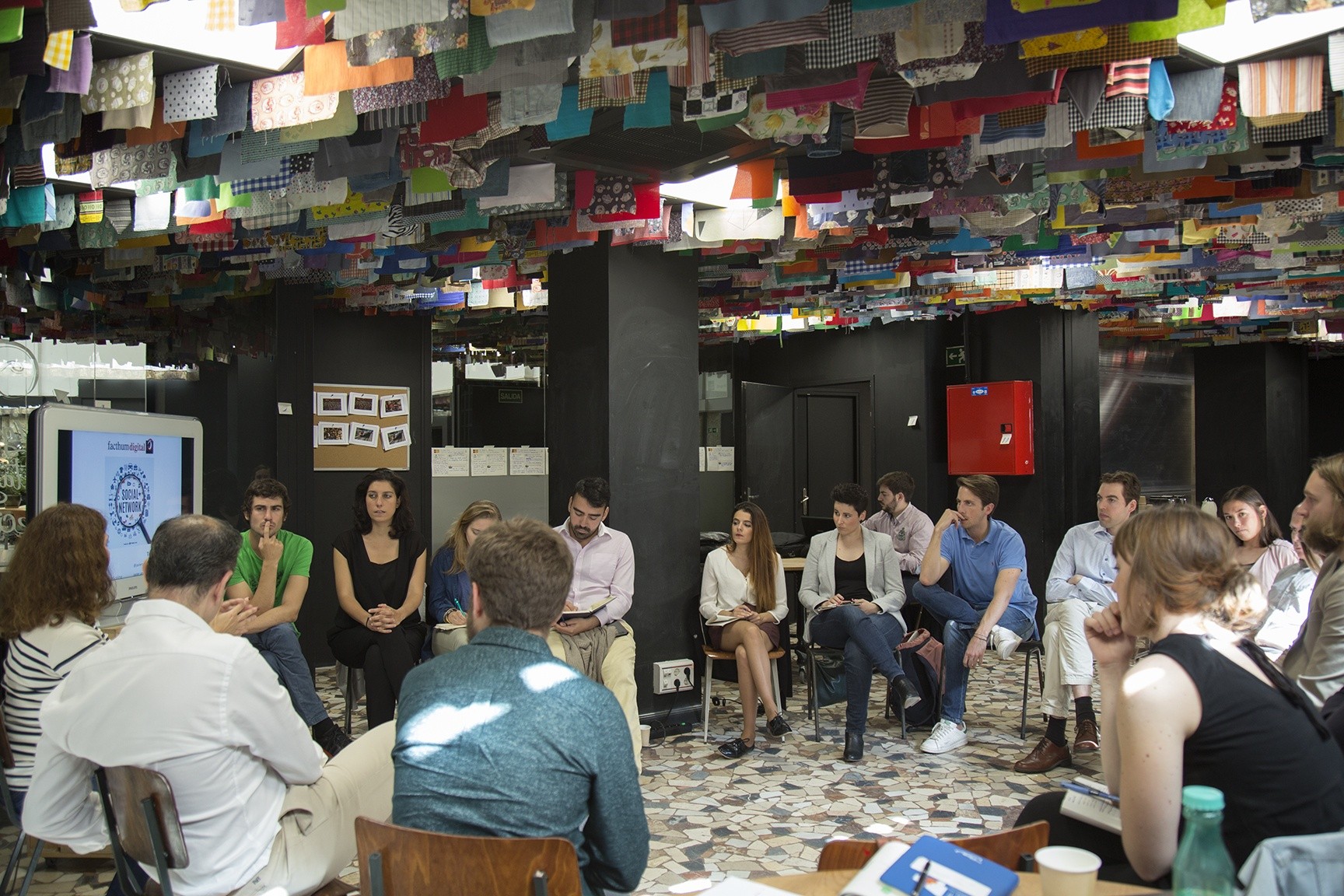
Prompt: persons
<box><xmin>1010</xmin><ymin>506</ymin><xmax>1342</xmax><ymax>890</ymax></box>
<box><xmin>391</xmin><ymin>518</ymin><xmax>650</xmax><ymax>895</ymax></box>
<box><xmin>549</xmin><ymin>477</ymin><xmax>643</xmax><ymax>779</ymax></box>
<box><xmin>430</xmin><ymin>498</ymin><xmax>504</xmax><ymax>656</ymax></box>
<box><xmin>225</xmin><ymin>478</ymin><xmax>353</xmax><ymax>759</ymax></box>
<box><xmin>1217</xmin><ymin>451</ymin><xmax>1343</xmax><ymax>746</ymax></box>
<box><xmin>1011</xmin><ymin>467</ymin><xmax>1142</xmax><ymax>773</ymax></box>
<box><xmin>859</xmin><ymin>469</ymin><xmax>936</xmax><ymax>608</ymax></box>
<box><xmin>799</xmin><ymin>482</ymin><xmax>922</xmax><ymax>762</ymax></box>
<box><xmin>22</xmin><ymin>513</ymin><xmax>397</xmax><ymax>895</ymax></box>
<box><xmin>911</xmin><ymin>474</ymin><xmax>1040</xmax><ymax>754</ymax></box>
<box><xmin>699</xmin><ymin>501</ymin><xmax>793</xmax><ymax>759</ymax></box>
<box><xmin>3</xmin><ymin>501</ymin><xmax>259</xmax><ymax>818</ymax></box>
<box><xmin>327</xmin><ymin>466</ymin><xmax>428</xmax><ymax>733</ymax></box>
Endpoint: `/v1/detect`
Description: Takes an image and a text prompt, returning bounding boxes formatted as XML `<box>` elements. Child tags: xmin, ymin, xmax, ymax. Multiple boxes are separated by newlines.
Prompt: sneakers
<box><xmin>990</xmin><ymin>625</ymin><xmax>1023</xmax><ymax>660</ymax></box>
<box><xmin>920</xmin><ymin>718</ymin><xmax>968</xmax><ymax>753</ymax></box>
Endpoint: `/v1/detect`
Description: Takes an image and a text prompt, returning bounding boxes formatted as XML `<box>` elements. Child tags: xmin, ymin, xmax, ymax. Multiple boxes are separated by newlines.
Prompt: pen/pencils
<box><xmin>910</xmin><ymin>858</ymin><xmax>931</xmax><ymax>896</ymax></box>
<box><xmin>1062</xmin><ymin>781</ymin><xmax>1121</xmax><ymax>803</ymax></box>
<box><xmin>454</xmin><ymin>598</ymin><xmax>467</xmax><ymax>618</ymax></box>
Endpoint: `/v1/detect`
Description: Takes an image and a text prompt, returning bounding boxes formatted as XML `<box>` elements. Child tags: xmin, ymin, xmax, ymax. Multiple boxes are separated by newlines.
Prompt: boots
<box><xmin>844</xmin><ymin>730</ymin><xmax>864</xmax><ymax>762</ymax></box>
<box><xmin>894</xmin><ymin>677</ymin><xmax>921</xmax><ymax>709</ymax></box>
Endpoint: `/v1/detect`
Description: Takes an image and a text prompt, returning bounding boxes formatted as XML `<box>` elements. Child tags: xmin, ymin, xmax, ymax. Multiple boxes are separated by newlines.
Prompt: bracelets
<box><xmin>365</xmin><ymin>617</ymin><xmax>370</xmax><ymax>628</ymax></box>
<box><xmin>973</xmin><ymin>633</ymin><xmax>988</xmax><ymax>641</ymax></box>
<box><xmin>445</xmin><ymin>608</ymin><xmax>458</xmax><ymax>624</ymax></box>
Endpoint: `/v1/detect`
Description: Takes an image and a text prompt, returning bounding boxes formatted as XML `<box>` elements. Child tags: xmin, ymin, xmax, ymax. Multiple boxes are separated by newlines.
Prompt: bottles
<box><xmin>1172</xmin><ymin>785</ymin><xmax>1235</xmax><ymax>896</ymax></box>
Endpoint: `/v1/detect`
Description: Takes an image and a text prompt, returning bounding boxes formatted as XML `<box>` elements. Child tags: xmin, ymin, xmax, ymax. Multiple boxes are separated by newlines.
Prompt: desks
<box><xmin>753</xmin><ymin>870</ymin><xmax>1176</xmax><ymax>896</ymax></box>
<box><xmin>781</xmin><ymin>558</ymin><xmax>812</xmax><ymax>684</ymax></box>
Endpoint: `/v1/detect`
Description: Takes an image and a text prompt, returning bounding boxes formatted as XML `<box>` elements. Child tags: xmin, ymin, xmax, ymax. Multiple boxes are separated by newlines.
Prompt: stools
<box><xmin>935</xmin><ymin>640</ymin><xmax>1048</xmax><ymax>739</ymax></box>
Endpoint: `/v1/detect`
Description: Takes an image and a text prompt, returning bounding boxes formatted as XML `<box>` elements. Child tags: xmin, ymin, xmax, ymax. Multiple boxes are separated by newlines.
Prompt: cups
<box><xmin>1034</xmin><ymin>846</ymin><xmax>1102</xmax><ymax>896</ymax></box>
<box><xmin>1138</xmin><ymin>496</ymin><xmax>1146</xmax><ymax>512</ymax></box>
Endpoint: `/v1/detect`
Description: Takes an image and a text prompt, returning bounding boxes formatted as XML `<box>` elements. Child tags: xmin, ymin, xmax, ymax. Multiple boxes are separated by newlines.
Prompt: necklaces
<box><xmin>1168</xmin><ymin>611</ymin><xmax>1205</xmax><ymax>637</ymax></box>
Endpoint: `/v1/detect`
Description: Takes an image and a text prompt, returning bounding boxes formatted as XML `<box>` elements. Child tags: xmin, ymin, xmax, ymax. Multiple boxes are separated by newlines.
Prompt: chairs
<box><xmin>696</xmin><ymin>591</ymin><xmax>786</xmax><ymax>744</ymax></box>
<box><xmin>354</xmin><ymin>816</ymin><xmax>580</xmax><ymax>896</ymax></box>
<box><xmin>818</xmin><ymin>820</ymin><xmax>1049</xmax><ymax>871</ymax></box>
<box><xmin>93</xmin><ymin>766</ymin><xmax>189</xmax><ymax>896</ymax></box>
<box><xmin>803</xmin><ymin>605</ymin><xmax>906</xmax><ymax>742</ymax></box>
<box><xmin>0</xmin><ymin>707</ymin><xmax>116</xmax><ymax>896</ymax></box>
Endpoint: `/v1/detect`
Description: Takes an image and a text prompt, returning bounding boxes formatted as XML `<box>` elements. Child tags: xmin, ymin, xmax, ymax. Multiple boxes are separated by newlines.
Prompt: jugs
<box><xmin>1201</xmin><ymin>497</ymin><xmax>1217</xmax><ymax>517</ymax></box>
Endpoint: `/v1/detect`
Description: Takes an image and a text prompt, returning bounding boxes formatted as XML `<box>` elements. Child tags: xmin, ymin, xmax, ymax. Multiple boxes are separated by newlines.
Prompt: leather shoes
<box><xmin>312</xmin><ymin>724</ymin><xmax>354</xmax><ymax>759</ymax></box>
<box><xmin>717</xmin><ymin>737</ymin><xmax>755</xmax><ymax>759</ymax></box>
<box><xmin>1015</xmin><ymin>737</ymin><xmax>1070</xmax><ymax>774</ymax></box>
<box><xmin>1073</xmin><ymin>719</ymin><xmax>1101</xmax><ymax>752</ymax></box>
<box><xmin>766</xmin><ymin>712</ymin><xmax>793</xmax><ymax>738</ymax></box>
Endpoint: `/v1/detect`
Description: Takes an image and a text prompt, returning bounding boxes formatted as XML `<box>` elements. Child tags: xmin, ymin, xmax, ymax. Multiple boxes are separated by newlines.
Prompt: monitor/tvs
<box><xmin>26</xmin><ymin>401</ymin><xmax>206</xmax><ymax>616</ymax></box>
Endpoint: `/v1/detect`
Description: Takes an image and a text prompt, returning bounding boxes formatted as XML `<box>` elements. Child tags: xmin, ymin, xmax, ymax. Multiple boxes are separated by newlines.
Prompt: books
<box><xmin>434</xmin><ymin>623</ymin><xmax>467</xmax><ymax>631</ymax></box>
<box><xmin>817</xmin><ymin>601</ymin><xmax>859</xmax><ymax>611</ymax></box>
<box><xmin>1060</xmin><ymin>776</ymin><xmax>1122</xmax><ymax>835</ymax></box>
<box><xmin>562</xmin><ymin>596</ymin><xmax>617</xmax><ymax>620</ymax></box>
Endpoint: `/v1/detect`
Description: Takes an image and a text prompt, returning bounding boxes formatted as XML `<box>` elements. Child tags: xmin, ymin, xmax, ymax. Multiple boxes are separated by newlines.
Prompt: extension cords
<box><xmin>648</xmin><ymin>722</ymin><xmax>693</xmax><ymax>739</ymax></box>
